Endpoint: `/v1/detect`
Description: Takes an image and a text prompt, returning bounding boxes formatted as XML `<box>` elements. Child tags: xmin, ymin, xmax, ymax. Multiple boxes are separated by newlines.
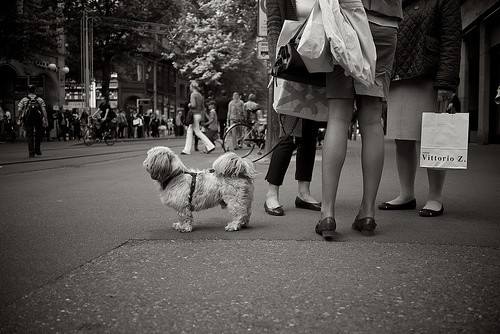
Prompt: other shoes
<box><xmin>420</xmin><ymin>204</ymin><xmax>444</xmax><ymax>216</ymax></box>
<box><xmin>207</xmin><ymin>148</ymin><xmax>215</xmax><ymax>153</ymax></box>
<box><xmin>378</xmin><ymin>198</ymin><xmax>416</xmax><ymax>210</ymax></box>
<box><xmin>180</xmin><ymin>152</ymin><xmax>186</xmax><ymax>154</ymax></box>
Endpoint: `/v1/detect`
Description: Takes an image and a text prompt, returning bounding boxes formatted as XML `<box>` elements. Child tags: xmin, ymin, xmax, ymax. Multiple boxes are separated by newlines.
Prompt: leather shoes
<box><xmin>315</xmin><ymin>217</ymin><xmax>336</xmax><ymax>237</ymax></box>
<box><xmin>295</xmin><ymin>196</ymin><xmax>322</xmax><ymax>211</ymax></box>
<box><xmin>352</xmin><ymin>215</ymin><xmax>377</xmax><ymax>236</ymax></box>
<box><xmin>263</xmin><ymin>200</ymin><xmax>284</xmax><ymax>216</ymax></box>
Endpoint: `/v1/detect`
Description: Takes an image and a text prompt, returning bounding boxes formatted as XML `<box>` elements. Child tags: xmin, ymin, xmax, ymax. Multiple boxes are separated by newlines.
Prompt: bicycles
<box><xmin>222</xmin><ymin>107</ymin><xmax>298</xmax><ymax>158</ymax></box>
<box><xmin>83</xmin><ymin>117</ymin><xmax>118</xmax><ymax>146</ymax></box>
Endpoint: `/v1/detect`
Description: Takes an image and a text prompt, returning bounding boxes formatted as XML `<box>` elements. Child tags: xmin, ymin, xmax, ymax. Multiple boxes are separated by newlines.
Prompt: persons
<box><xmin>90</xmin><ymin>97</ymin><xmax>115</xmax><ymax>142</ymax></box>
<box><xmin>181</xmin><ymin>80</ymin><xmax>261</xmax><ymax>155</ymax></box>
<box><xmin>264</xmin><ymin>0</ymin><xmax>463</xmax><ymax>238</ymax></box>
<box><xmin>18</xmin><ymin>85</ymin><xmax>47</xmax><ymax>158</ymax></box>
<box><xmin>112</xmin><ymin>107</ymin><xmax>185</xmax><ymax>139</ymax></box>
<box><xmin>0</xmin><ymin>103</ymin><xmax>89</xmax><ymax>141</ymax></box>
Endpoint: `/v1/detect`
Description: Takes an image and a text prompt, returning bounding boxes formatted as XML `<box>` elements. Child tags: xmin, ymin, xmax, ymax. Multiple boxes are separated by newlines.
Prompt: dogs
<box><xmin>143</xmin><ymin>146</ymin><xmax>258</xmax><ymax>233</ymax></box>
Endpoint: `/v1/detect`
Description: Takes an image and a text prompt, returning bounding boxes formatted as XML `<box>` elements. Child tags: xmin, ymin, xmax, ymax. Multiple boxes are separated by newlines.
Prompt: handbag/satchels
<box><xmin>266</xmin><ymin>0</ymin><xmax>334</xmax><ymax>122</ymax></box>
<box><xmin>420</xmin><ymin>99</ymin><xmax>469</xmax><ymax>169</ymax></box>
<box><xmin>185</xmin><ymin>106</ymin><xmax>194</xmax><ymax>126</ymax></box>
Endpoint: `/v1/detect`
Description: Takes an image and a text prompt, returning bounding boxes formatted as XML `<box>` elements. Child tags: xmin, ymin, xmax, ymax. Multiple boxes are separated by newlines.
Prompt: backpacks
<box><xmin>24</xmin><ymin>94</ymin><xmax>43</xmax><ymax>128</ymax></box>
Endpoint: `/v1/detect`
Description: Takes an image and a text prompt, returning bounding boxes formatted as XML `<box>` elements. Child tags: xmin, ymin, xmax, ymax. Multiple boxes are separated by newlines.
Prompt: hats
<box><xmin>206</xmin><ymin>100</ymin><xmax>216</xmax><ymax>105</ymax></box>
